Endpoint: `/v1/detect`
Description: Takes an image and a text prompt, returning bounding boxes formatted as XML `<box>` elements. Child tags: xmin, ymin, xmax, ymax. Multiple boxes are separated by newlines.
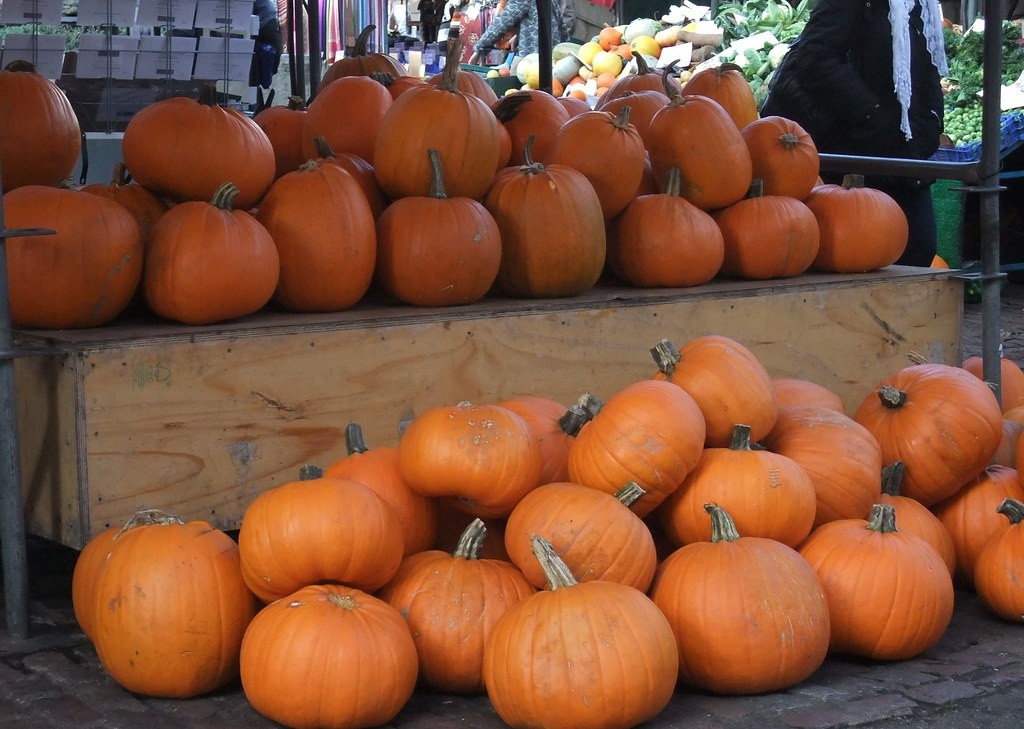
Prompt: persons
<box><xmin>505</xmin><ymin>0</ymin><xmax>577</xmax><ymax>52</ymax></box>
<box><xmin>760</xmin><ymin>0</ymin><xmax>942</xmax><ymax>268</ymax></box>
<box><xmin>468</xmin><ymin>0</ymin><xmax>569</xmax><ymax>65</ymax></box>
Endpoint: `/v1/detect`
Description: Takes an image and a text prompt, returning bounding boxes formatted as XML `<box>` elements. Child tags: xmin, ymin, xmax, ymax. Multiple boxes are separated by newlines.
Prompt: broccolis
<box><xmin>714</xmin><ymin>0</ymin><xmax>819</xmax><ymax>114</ymax></box>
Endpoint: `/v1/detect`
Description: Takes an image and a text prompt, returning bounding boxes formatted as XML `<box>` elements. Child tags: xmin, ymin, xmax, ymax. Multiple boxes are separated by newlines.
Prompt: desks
<box><xmin>25</xmin><ymin>265</ymin><xmax>962</xmax><ymax>561</ymax></box>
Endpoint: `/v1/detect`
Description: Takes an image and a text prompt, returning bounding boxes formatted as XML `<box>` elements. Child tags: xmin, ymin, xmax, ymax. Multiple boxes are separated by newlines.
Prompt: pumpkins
<box><xmin>71</xmin><ymin>331</ymin><xmax>1024</xmax><ymax>729</ymax></box>
<box><xmin>1</xmin><ymin>22</ymin><xmax>951</xmax><ymax>332</ymax></box>
